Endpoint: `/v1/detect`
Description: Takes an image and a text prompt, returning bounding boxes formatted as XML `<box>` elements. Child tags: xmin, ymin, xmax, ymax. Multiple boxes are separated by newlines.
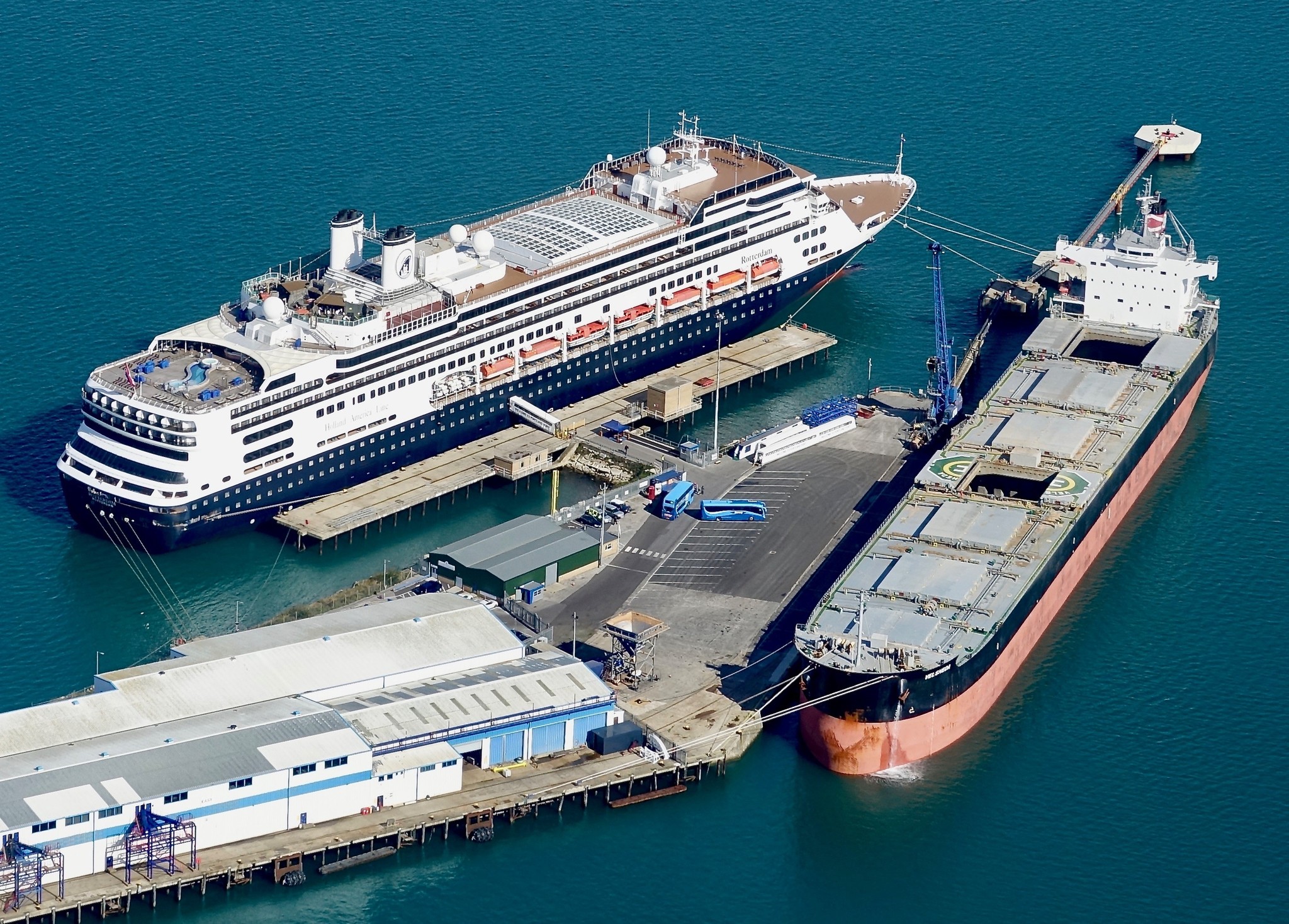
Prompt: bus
<box><xmin>662</xmin><ymin>480</ymin><xmax>694</xmax><ymax>521</ymax></box>
<box><xmin>701</xmin><ymin>500</ymin><xmax>768</xmax><ymax>522</ymax></box>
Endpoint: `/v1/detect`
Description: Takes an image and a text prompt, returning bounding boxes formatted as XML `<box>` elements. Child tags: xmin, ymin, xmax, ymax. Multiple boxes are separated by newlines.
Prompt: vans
<box><xmin>418</xmin><ymin>581</ymin><xmax>443</xmax><ymax>593</ymax></box>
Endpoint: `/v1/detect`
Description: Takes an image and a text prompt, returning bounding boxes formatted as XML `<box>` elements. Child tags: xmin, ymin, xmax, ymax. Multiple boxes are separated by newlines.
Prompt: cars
<box><xmin>446</xmin><ymin>586</ymin><xmax>499</xmax><ymax>609</ymax></box>
<box><xmin>610</xmin><ymin>499</ymin><xmax>631</xmax><ymax>514</ymax></box>
<box><xmin>580</xmin><ymin>515</ymin><xmax>602</xmax><ymax>529</ymax></box>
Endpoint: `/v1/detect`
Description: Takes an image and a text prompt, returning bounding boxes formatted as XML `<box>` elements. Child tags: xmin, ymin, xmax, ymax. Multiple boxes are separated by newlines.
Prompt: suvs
<box><xmin>602</xmin><ymin>503</ymin><xmax>624</xmax><ymax>519</ymax></box>
<box><xmin>589</xmin><ymin>507</ymin><xmax>612</xmax><ymax>524</ymax></box>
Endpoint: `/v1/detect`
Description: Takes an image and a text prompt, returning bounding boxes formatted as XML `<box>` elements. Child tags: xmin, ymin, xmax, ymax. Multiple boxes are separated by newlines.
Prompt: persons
<box><xmin>564</xmin><ymin>430</ymin><xmax>567</xmax><ymax>439</ymax></box>
<box><xmin>697</xmin><ymin>487</ymin><xmax>701</xmax><ymax>495</ymax></box>
<box><xmin>661</xmin><ymin>455</ymin><xmax>666</xmax><ymax>463</ymax></box>
<box><xmin>361</xmin><ymin>303</ymin><xmax>368</xmax><ymax>317</ymax></box>
<box><xmin>556</xmin><ymin>426</ymin><xmax>559</xmax><ymax>436</ymax></box>
<box><xmin>624</xmin><ymin>444</ymin><xmax>629</xmax><ymax>454</ymax></box>
<box><xmin>701</xmin><ymin>486</ymin><xmax>704</xmax><ymax>495</ymax></box>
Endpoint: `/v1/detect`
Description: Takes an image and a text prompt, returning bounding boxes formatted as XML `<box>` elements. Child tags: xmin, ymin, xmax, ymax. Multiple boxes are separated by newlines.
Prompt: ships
<box><xmin>56</xmin><ymin>109</ymin><xmax>918</xmax><ymax>554</ymax></box>
<box><xmin>791</xmin><ymin>174</ymin><xmax>1218</xmax><ymax>781</ymax></box>
